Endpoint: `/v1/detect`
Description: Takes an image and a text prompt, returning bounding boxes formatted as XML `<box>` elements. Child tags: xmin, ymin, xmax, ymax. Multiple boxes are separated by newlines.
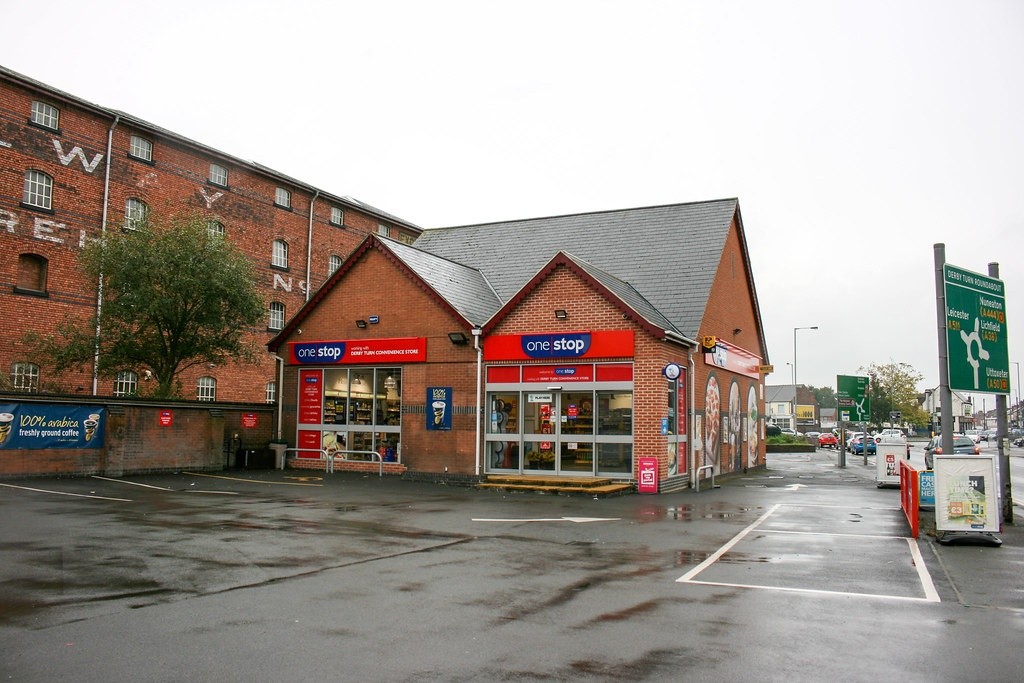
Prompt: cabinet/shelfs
<box><xmin>324</xmin><ymin>406</ymin><xmax>400</xmax><ymax>462</ymax></box>
<box><xmin>507</xmin><ymin>415</ymin><xmax>631</xmax><ymax>466</ymax></box>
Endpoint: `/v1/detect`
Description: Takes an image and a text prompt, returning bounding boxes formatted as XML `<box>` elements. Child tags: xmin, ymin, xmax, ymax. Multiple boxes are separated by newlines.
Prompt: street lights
<box><xmin>786</xmin><ymin>362</ymin><xmax>795</xmax><ymax>413</ymax></box>
<box><xmin>793</xmin><ymin>326</ymin><xmax>818</xmax><ymax>438</ymax></box>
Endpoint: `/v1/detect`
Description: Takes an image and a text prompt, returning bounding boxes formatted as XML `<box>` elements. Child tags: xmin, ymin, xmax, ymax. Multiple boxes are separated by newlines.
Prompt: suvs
<box><xmin>925</xmin><ymin>432</ymin><xmax>979</xmax><ymax>470</ymax></box>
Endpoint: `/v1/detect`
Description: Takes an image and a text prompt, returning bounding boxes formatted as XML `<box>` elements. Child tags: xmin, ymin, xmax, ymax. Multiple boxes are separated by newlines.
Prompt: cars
<box><xmin>963</xmin><ymin>426</ymin><xmax>1024</xmax><ymax>446</ymax></box>
<box><xmin>805</xmin><ymin>432</ymin><xmax>838</xmax><ymax>449</ymax></box>
<box><xmin>832</xmin><ymin>428</ymin><xmax>914</xmax><ymax>459</ymax></box>
<box><xmin>782</xmin><ymin>428</ymin><xmax>803</xmax><ymax>438</ymax></box>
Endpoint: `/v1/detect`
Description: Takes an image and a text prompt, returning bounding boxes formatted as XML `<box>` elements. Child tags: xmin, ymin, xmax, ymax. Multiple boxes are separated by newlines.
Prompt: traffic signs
<box><xmin>837</xmin><ymin>374</ymin><xmax>870</xmax><ymax>422</ymax></box>
<box><xmin>943</xmin><ymin>263</ymin><xmax>1010</xmax><ymax>395</ymax></box>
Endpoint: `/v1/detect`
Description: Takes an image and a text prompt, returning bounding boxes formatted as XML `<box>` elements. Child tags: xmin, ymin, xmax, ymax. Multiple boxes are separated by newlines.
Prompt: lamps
<box><xmin>554</xmin><ymin>309</ymin><xmax>566</xmax><ymax>318</ymax></box>
<box><xmin>448</xmin><ymin>332</ymin><xmax>470</xmax><ymax>345</ymax></box>
<box><xmin>735</xmin><ymin>328</ymin><xmax>742</xmax><ymax>334</ymax></box>
<box><xmin>356</xmin><ymin>320</ymin><xmax>367</xmax><ymax>328</ymax></box>
